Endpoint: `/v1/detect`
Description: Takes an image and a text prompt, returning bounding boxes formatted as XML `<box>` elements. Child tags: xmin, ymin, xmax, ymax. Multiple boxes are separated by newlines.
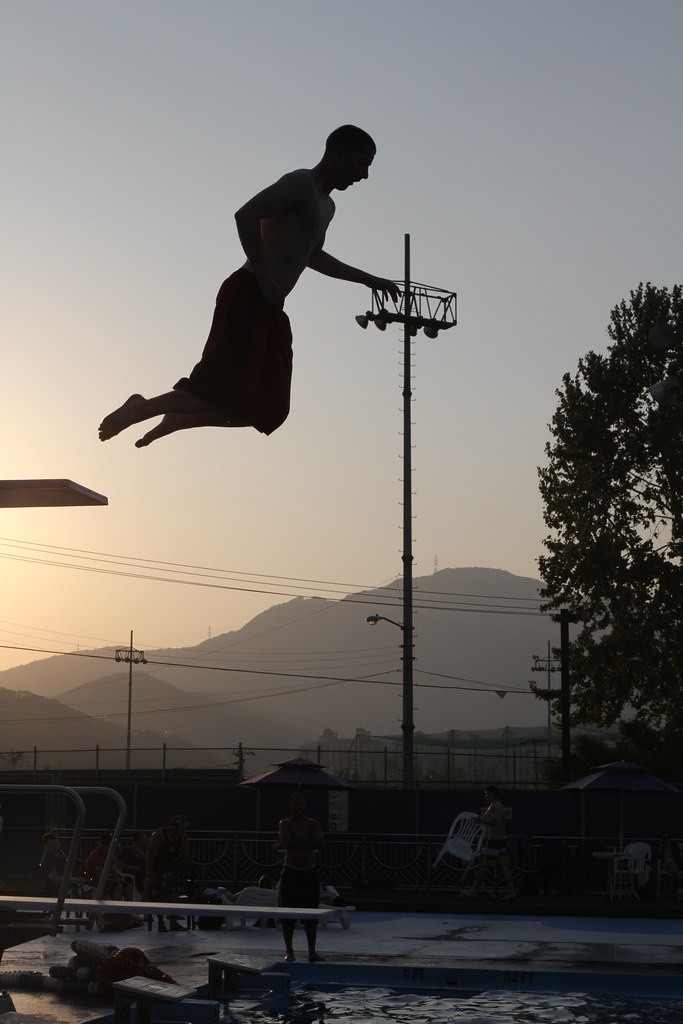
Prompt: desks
<box><xmin>593</xmin><ymin>852</ymin><xmax>641</xmax><ymax>901</ymax></box>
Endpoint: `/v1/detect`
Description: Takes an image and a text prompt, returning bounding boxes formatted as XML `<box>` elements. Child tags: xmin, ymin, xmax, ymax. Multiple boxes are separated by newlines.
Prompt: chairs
<box><xmin>623</xmin><ymin>842</ymin><xmax>652</xmax><ymax>875</ymax></box>
<box><xmin>39</xmin><ymin>863</ymin><xmax>82</xmax><ymax>918</ymax></box>
<box><xmin>220</xmin><ymin>887</ymin><xmax>280</xmax><ymax>932</ymax></box>
<box><xmin>433</xmin><ymin>811</ymin><xmax>487</xmax><ymax>883</ymax></box>
<box><xmin>78</xmin><ymin>861</ymin><xmax>136</xmax><ymax>901</ymax></box>
<box><xmin>655</xmin><ymin>843</ymin><xmax>683</xmax><ymax>896</ymax></box>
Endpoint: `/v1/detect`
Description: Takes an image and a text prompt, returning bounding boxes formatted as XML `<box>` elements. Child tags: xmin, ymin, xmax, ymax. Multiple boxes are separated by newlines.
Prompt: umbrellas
<box><xmin>560</xmin><ymin>760</ymin><xmax>679</xmax><ymax>855</ymax></box>
<box><xmin>235</xmin><ymin>759</ymin><xmax>364</xmax><ymax>793</ymax></box>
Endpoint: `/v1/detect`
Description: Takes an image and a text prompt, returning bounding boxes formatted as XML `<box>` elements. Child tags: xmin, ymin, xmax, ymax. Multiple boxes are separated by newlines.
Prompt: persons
<box><xmin>317</xmin><ymin>892</ymin><xmax>347</xmax><ymax>908</ymax></box>
<box><xmin>459</xmin><ymin>785</ymin><xmax>520</xmax><ymax>902</ymax></box>
<box><xmin>39</xmin><ymin>832</ymin><xmax>143</xmax><ymax>930</ymax></box>
<box><xmin>99</xmin><ymin>125</ymin><xmax>378</xmax><ymax>448</ymax></box>
<box><xmin>141</xmin><ymin>813</ymin><xmax>195</xmax><ymax>931</ymax></box>
<box><xmin>114</xmin><ymin>830</ymin><xmax>148</xmax><ymax>893</ymax></box>
<box><xmin>220</xmin><ymin>873</ymin><xmax>273</xmax><ymax>903</ymax></box>
<box><xmin>657</xmin><ymin>831</ymin><xmax>683</xmax><ymax>881</ymax></box>
<box><xmin>81</xmin><ymin>832</ymin><xmax>145</xmax><ymax>932</ymax></box>
<box><xmin>277</xmin><ymin>792</ymin><xmax>328</xmax><ymax>962</ymax></box>
